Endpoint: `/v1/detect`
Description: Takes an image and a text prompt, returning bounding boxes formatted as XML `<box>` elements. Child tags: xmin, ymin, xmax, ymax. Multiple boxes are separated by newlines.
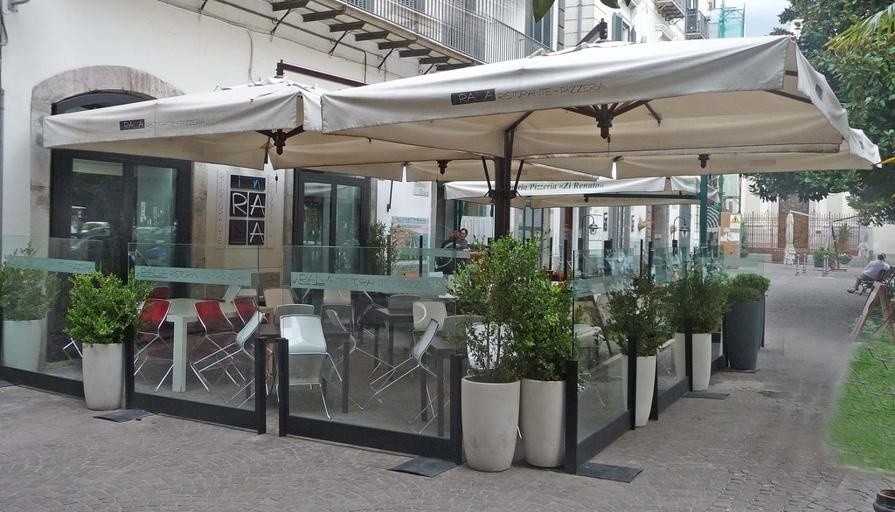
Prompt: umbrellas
<box><xmin>42</xmin><ymin>31</ymin><xmax>884</xmax><ymax>274</ymax></box>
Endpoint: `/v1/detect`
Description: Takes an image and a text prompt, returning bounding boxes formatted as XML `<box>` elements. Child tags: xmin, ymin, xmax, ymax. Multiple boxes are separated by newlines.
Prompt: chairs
<box><xmin>859</xmin><ymin>264</ymin><xmax>895</xmax><ymax>296</ymax></box>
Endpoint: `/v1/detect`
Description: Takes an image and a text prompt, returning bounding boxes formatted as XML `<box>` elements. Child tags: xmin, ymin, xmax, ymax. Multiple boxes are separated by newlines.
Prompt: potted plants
<box><xmin>666</xmin><ymin>262</ymin><xmax>736</xmax><ymax>392</ymax></box>
<box><xmin>836</xmin><ymin>223</ymin><xmax>854</xmax><ymax>248</ymax></box>
<box><xmin>513</xmin><ymin>277</ymin><xmax>577</xmax><ymax>468</ymax></box>
<box><xmin>62</xmin><ymin>268</ymin><xmax>157</xmax><ymax>411</ymax></box>
<box><xmin>729</xmin><ymin>273</ymin><xmax>770</xmax><ymax>347</ymax></box>
<box><xmin>725</xmin><ymin>288</ymin><xmax>764</xmax><ymax>369</ymax></box>
<box><xmin>441</xmin><ymin>235</ymin><xmax>545</xmax><ymax>474</ymax></box>
<box><xmin>605</xmin><ymin>271</ymin><xmax>676</xmax><ymax>428</ymax></box>
<box><xmin>1</xmin><ymin>239</ymin><xmax>63</xmax><ymax>371</ymax></box>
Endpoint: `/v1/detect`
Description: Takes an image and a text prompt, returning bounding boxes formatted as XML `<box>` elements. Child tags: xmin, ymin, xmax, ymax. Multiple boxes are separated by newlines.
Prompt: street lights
<box><xmin>580</xmin><ymin>215</ymin><xmax>599</xmax><ymax>279</ymax></box>
<box><xmin>672</xmin><ymin>216</ymin><xmax>691</xmax><ymax>268</ymax></box>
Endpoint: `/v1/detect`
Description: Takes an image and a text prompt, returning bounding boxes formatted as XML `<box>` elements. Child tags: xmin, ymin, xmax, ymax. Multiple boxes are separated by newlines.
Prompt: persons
<box><xmin>457</xmin><ymin>228</ymin><xmax>472</xmax><ymax>271</ymax></box>
<box><xmin>437</xmin><ymin>232</ymin><xmax>461</xmax><ymax>275</ymax></box>
<box><xmin>847</xmin><ymin>253</ymin><xmax>890</xmax><ymax>293</ymax></box>
